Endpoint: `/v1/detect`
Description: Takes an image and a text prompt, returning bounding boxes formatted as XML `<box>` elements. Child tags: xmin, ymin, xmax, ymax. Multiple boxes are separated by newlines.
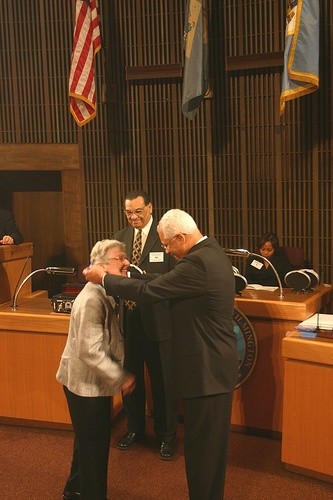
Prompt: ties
<box><xmin>124</xmin><ymin>228</ymin><xmax>142</xmax><ymax>311</ymax></box>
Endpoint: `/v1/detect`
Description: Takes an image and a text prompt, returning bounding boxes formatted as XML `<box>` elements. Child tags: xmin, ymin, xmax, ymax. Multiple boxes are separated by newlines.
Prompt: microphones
<box><xmin>221</xmin><ymin>248</ymin><xmax>284</xmax><ymax>298</ymax></box>
<box><xmin>13</xmin><ymin>266</ymin><xmax>75</xmax><ymax>310</ymax></box>
<box><xmin>10</xmin><ymin>239</ymin><xmax>36</xmax><ymax>306</ymax></box>
<box><xmin>308</xmin><ymin>267</ymin><xmax>332</xmax><ymax>338</ymax></box>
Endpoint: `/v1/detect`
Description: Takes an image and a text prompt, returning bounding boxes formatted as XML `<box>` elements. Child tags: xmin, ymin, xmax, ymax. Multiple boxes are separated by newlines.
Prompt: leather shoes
<box><xmin>159</xmin><ymin>441</ymin><xmax>172</xmax><ymax>460</ymax></box>
<box><xmin>117</xmin><ymin>430</ymin><xmax>144</xmax><ymax>450</ymax></box>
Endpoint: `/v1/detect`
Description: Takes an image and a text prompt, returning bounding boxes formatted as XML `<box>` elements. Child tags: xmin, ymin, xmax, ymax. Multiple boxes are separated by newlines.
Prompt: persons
<box><xmin>246</xmin><ymin>232</ymin><xmax>289</xmax><ymax>286</ymax></box>
<box><xmin>82</xmin><ymin>209</ymin><xmax>237</xmax><ymax>499</ymax></box>
<box><xmin>112</xmin><ymin>190</ymin><xmax>176</xmax><ymax>459</ymax></box>
<box><xmin>55</xmin><ymin>240</ymin><xmax>136</xmax><ymax>499</ymax></box>
<box><xmin>0</xmin><ymin>209</ymin><xmax>23</xmax><ymax>245</ymax></box>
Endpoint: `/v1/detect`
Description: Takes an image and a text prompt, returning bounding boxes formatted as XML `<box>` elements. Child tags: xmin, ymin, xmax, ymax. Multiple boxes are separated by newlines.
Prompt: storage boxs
<box><xmin>51</xmin><ymin>294</ymin><xmax>77</xmax><ymax>314</ymax></box>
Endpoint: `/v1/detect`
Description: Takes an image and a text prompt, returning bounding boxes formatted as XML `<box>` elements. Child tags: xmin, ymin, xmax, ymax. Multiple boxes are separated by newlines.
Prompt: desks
<box><xmin>0</xmin><ymin>281</ymin><xmax>333</xmax><ymax>483</ymax></box>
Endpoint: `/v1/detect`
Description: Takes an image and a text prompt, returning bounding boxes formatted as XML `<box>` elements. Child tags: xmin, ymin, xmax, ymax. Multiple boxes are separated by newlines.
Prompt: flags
<box><xmin>280</xmin><ymin>0</ymin><xmax>320</xmax><ymax>114</ymax></box>
<box><xmin>181</xmin><ymin>0</ymin><xmax>214</xmax><ymax>120</ymax></box>
<box><xmin>67</xmin><ymin>0</ymin><xmax>101</xmax><ymax>126</ymax></box>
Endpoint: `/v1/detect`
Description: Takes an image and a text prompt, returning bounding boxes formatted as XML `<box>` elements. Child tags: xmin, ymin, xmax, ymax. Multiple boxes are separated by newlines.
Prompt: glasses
<box><xmin>103</xmin><ymin>256</ymin><xmax>130</xmax><ymax>262</ymax></box>
<box><xmin>160</xmin><ymin>233</ymin><xmax>187</xmax><ymax>248</ymax></box>
<box><xmin>123</xmin><ymin>204</ymin><xmax>147</xmax><ymax>216</ymax></box>
